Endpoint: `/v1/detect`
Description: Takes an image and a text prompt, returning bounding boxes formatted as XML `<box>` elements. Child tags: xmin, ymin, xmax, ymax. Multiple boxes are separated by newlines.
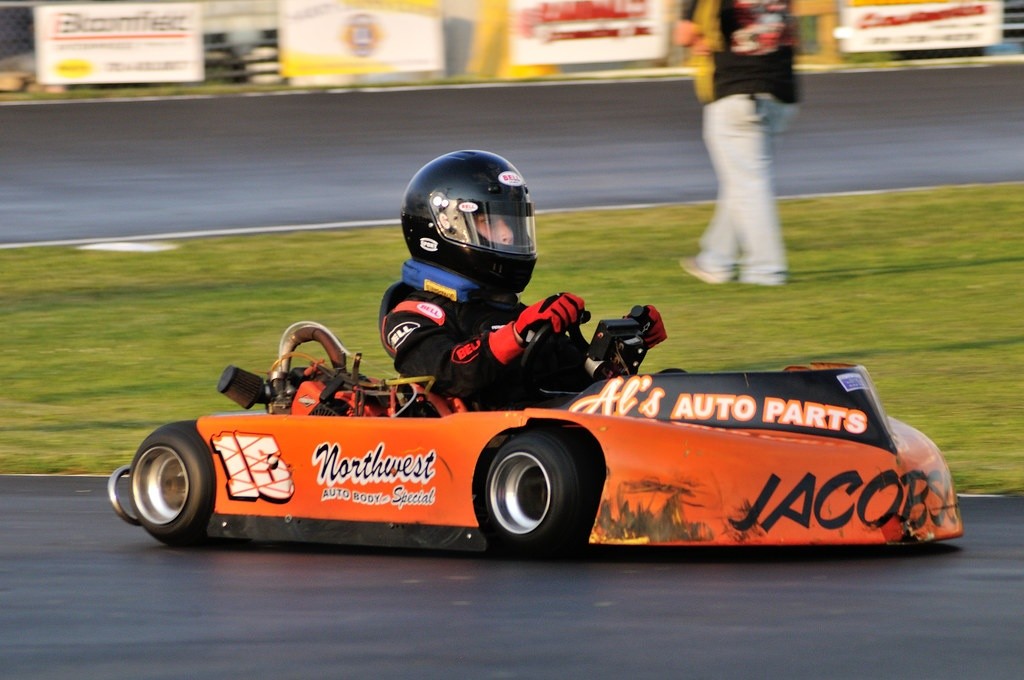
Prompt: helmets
<box><xmin>398</xmin><ymin>150</ymin><xmax>540</xmax><ymax>294</ymax></box>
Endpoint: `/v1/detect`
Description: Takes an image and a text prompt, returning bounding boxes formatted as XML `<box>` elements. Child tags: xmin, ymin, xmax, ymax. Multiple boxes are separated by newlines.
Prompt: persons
<box><xmin>672</xmin><ymin>0</ymin><xmax>800</xmax><ymax>286</ymax></box>
<box><xmin>381</xmin><ymin>150</ymin><xmax>669</xmax><ymax>409</ymax></box>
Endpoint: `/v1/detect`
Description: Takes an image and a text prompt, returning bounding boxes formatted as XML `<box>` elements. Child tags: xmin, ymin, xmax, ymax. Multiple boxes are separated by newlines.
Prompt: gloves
<box><xmin>624</xmin><ymin>303</ymin><xmax>667</xmax><ymax>350</ymax></box>
<box><xmin>515</xmin><ymin>292</ymin><xmax>587</xmax><ymax>347</ymax></box>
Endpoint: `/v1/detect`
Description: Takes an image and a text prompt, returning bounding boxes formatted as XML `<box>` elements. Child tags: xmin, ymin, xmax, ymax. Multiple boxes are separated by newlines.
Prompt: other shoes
<box><xmin>676</xmin><ymin>255</ymin><xmax>734</xmax><ymax>287</ymax></box>
<box><xmin>738</xmin><ymin>264</ymin><xmax>790</xmax><ymax>289</ymax></box>
